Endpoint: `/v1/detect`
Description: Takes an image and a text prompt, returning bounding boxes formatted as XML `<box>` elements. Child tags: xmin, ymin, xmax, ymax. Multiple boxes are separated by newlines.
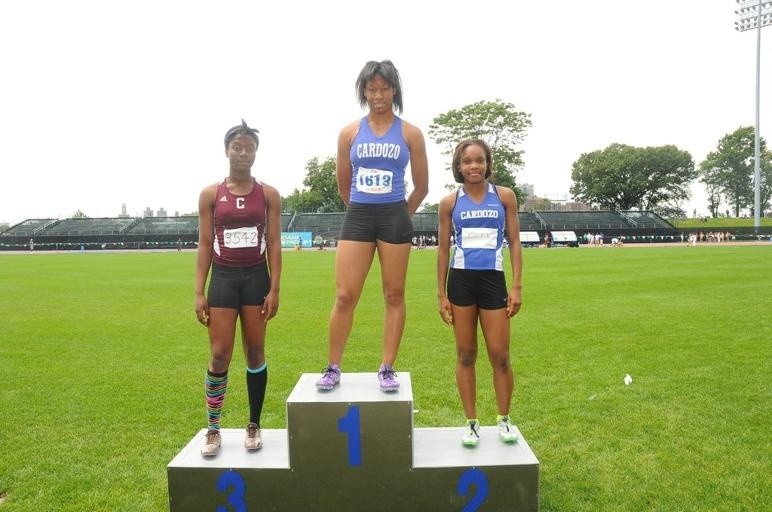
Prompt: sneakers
<box><xmin>315</xmin><ymin>364</ymin><xmax>341</xmax><ymax>390</ymax></box>
<box><xmin>377</xmin><ymin>366</ymin><xmax>399</xmax><ymax>391</ymax></box>
<box><xmin>244</xmin><ymin>422</ymin><xmax>263</xmax><ymax>449</ymax></box>
<box><xmin>496</xmin><ymin>414</ymin><xmax>518</xmax><ymax>442</ymax></box>
<box><xmin>201</xmin><ymin>430</ymin><xmax>222</xmax><ymax>456</ymax></box>
<box><xmin>462</xmin><ymin>419</ymin><xmax>480</xmax><ymax>446</ymax></box>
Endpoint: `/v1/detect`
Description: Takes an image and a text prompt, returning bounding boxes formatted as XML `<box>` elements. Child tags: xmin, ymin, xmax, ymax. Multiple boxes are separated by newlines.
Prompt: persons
<box><xmin>535</xmin><ymin>231</ymin><xmax>627</xmax><ymax>246</ymax></box>
<box><xmin>683</xmin><ymin>230</ymin><xmax>733</xmax><ymax>244</ymax></box>
<box><xmin>435</xmin><ymin>139</ymin><xmax>523</xmax><ymax>444</ymax></box>
<box><xmin>315</xmin><ymin>58</ymin><xmax>430</xmax><ymax>394</ymax></box>
<box><xmin>408</xmin><ymin>233</ymin><xmax>437</xmax><ymax>250</ymax></box>
<box><xmin>195</xmin><ymin>118</ymin><xmax>284</xmax><ymax>458</ymax></box>
